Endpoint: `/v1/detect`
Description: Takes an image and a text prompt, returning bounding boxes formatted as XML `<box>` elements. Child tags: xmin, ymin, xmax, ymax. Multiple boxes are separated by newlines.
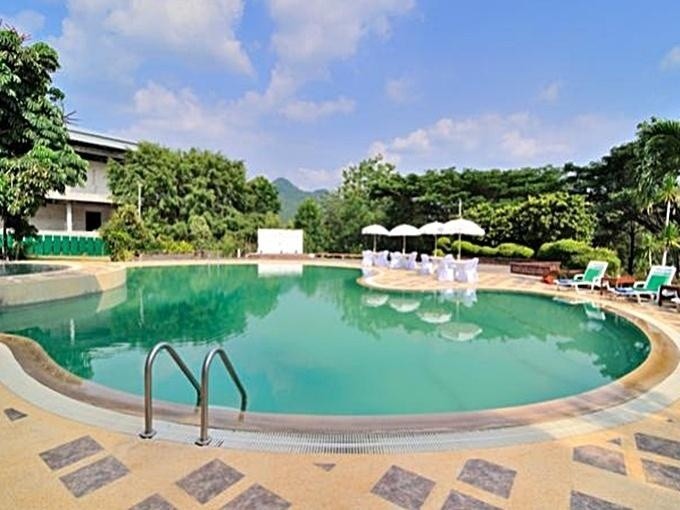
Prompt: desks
<box><xmin>658</xmin><ymin>283</ymin><xmax>680</xmax><ymax>307</ymax></box>
<box><xmin>557</xmin><ymin>269</ymin><xmax>583</xmax><ymax>280</ymax></box>
<box><xmin>600</xmin><ymin>275</ymin><xmax>635</xmax><ymax>296</ymax></box>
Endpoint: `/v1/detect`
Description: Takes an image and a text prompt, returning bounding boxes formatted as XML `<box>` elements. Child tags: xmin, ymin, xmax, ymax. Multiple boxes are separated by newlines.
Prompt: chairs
<box><xmin>554</xmin><ymin>261</ymin><xmax>608</xmax><ymax>293</ymax></box>
<box><xmin>611</xmin><ymin>266</ymin><xmax>673</xmax><ymax>303</ymax></box>
<box><xmin>360</xmin><ymin>249</ymin><xmax>479</xmax><ymax>285</ymax></box>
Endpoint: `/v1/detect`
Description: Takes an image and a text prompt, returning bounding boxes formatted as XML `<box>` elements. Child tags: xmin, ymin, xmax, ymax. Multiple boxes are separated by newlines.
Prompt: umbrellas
<box><xmin>441</xmin><ymin>216</ymin><xmax>485</xmax><ymax>260</ymax></box>
<box><xmin>389</xmin><ymin>223</ymin><xmax>418</xmax><ymax>254</ymax></box>
<box><xmin>418</xmin><ymin>221</ymin><xmax>444</xmax><ymax>257</ymax></box>
<box><xmin>440</xmin><ymin>296</ymin><xmax>482</xmax><ymax>341</ymax></box>
<box><xmin>362</xmin><ymin>287</ymin><xmax>389</xmax><ymax>307</ymax></box>
<box><xmin>416</xmin><ymin>293</ymin><xmax>451</xmax><ymax>323</ymax></box>
<box><xmin>388</xmin><ymin>291</ymin><xmax>420</xmax><ymax>312</ymax></box>
<box><xmin>362</xmin><ymin>225</ymin><xmax>389</xmax><ymax>252</ymax></box>
